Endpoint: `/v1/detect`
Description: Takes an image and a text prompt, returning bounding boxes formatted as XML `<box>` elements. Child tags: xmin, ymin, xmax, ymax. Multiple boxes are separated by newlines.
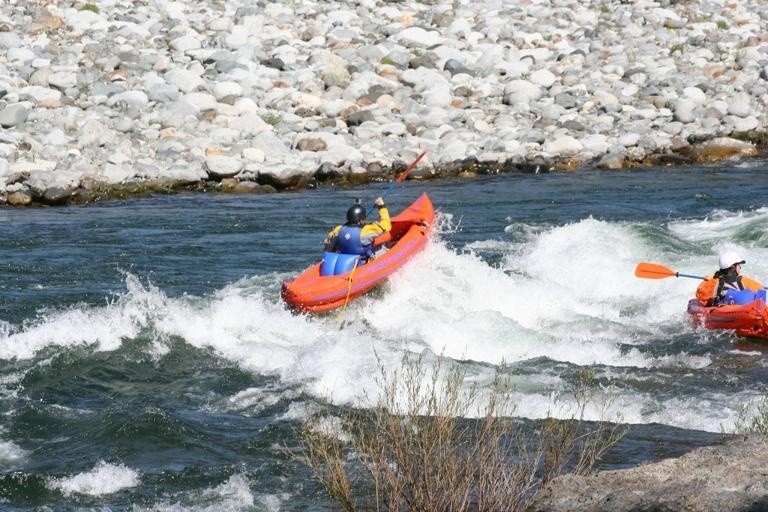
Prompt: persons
<box><xmin>706</xmin><ymin>252</ymin><xmax>747</xmax><ymax>308</ymax></box>
<box><xmin>324</xmin><ymin>197</ymin><xmax>392</xmax><ymax>263</ymax></box>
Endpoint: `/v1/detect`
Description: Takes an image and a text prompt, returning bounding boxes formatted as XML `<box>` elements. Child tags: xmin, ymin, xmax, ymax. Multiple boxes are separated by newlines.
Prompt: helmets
<box><xmin>719</xmin><ymin>252</ymin><xmax>746</xmax><ymax>271</ymax></box>
<box><xmin>346</xmin><ymin>204</ymin><xmax>367</xmax><ymax>223</ymax></box>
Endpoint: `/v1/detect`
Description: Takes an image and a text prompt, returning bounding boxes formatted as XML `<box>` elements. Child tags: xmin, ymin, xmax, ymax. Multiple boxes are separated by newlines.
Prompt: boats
<box><xmin>687</xmin><ymin>272</ymin><xmax>767</xmax><ymax>338</ymax></box>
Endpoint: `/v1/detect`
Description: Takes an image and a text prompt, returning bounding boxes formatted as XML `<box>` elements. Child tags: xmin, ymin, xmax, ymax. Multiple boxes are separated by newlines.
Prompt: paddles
<box><xmin>635</xmin><ymin>262</ymin><xmax>707</xmax><ymax>280</ymax></box>
<box><xmin>368</xmin><ymin>148</ymin><xmax>428</xmax><ymax>220</ymax></box>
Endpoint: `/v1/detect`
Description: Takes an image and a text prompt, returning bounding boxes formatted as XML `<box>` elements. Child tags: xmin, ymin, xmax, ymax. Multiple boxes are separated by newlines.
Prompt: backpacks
<box><xmin>695</xmin><ymin>275</ymin><xmax>723</xmax><ymax>308</ymax></box>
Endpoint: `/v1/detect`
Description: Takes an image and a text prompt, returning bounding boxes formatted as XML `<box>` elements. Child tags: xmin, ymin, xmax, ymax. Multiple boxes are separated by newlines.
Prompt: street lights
<box><xmin>279</xmin><ymin>191</ymin><xmax>436</xmax><ymax>315</ymax></box>
